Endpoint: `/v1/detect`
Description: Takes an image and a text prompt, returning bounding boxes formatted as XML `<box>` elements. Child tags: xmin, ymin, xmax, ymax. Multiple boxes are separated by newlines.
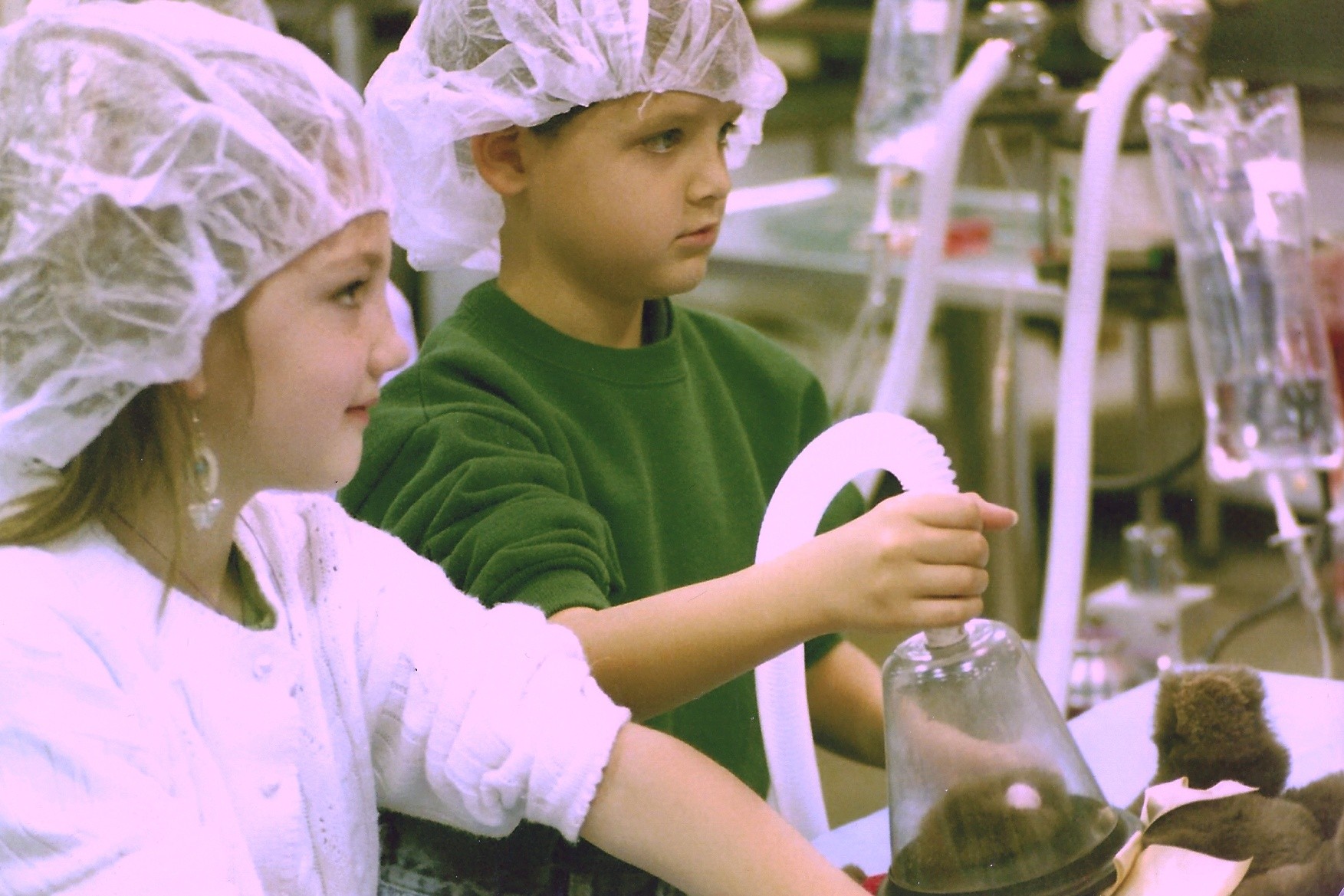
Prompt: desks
<box><xmin>826</xmin><ymin>653</ymin><xmax>1344</xmax><ymax>876</ymax></box>
<box><xmin>711</xmin><ymin>159</ymin><xmax>1075</xmax><ymax>602</ymax></box>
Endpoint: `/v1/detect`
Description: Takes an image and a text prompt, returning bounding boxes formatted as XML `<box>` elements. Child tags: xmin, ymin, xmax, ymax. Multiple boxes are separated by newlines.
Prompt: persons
<box><xmin>335</xmin><ymin>0</ymin><xmax>1019</xmax><ymax>896</ymax></box>
<box><xmin>0</xmin><ymin>0</ymin><xmax>871</xmax><ymax>896</ymax></box>
